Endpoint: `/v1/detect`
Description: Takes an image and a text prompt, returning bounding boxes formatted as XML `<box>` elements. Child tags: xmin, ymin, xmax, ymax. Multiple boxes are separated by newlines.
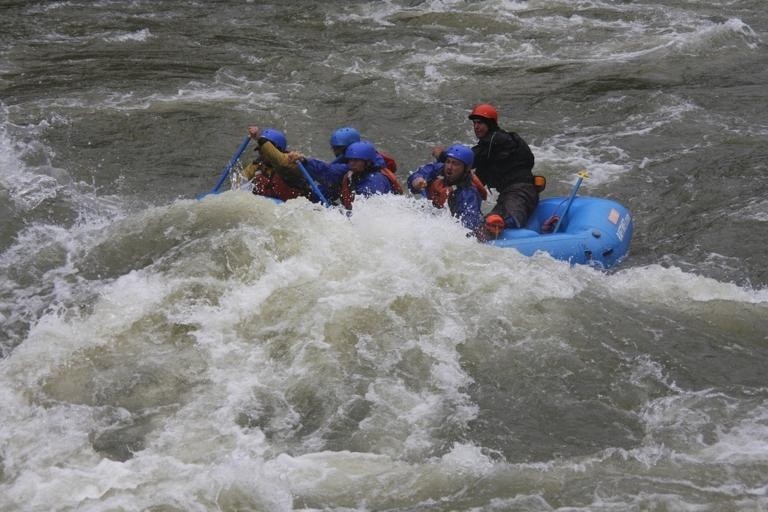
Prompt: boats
<box><xmin>194</xmin><ymin>197</ymin><xmax>633</xmax><ymax>269</ymax></box>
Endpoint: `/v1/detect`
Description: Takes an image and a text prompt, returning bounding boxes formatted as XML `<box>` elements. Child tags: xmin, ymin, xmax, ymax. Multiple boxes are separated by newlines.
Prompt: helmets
<box><xmin>258</xmin><ymin>105</ymin><xmax>497</xmax><ymax>164</ymax></box>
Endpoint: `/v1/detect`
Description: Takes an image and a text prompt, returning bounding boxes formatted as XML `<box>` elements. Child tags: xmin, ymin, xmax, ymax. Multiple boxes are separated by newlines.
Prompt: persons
<box><xmin>287</xmin><ymin>140</ymin><xmax>403</xmax><ymax>209</ymax></box>
<box><xmin>432</xmin><ymin>104</ymin><xmax>539</xmax><ymax>228</ymax></box>
<box><xmin>227</xmin><ymin>126</ymin><xmax>308</xmax><ymax>200</ymax></box>
<box><xmin>407</xmin><ymin>146</ymin><xmax>497</xmax><ymax>242</ymax></box>
<box><xmin>309</xmin><ymin>126</ymin><xmax>397</xmax><ymax>204</ymax></box>
